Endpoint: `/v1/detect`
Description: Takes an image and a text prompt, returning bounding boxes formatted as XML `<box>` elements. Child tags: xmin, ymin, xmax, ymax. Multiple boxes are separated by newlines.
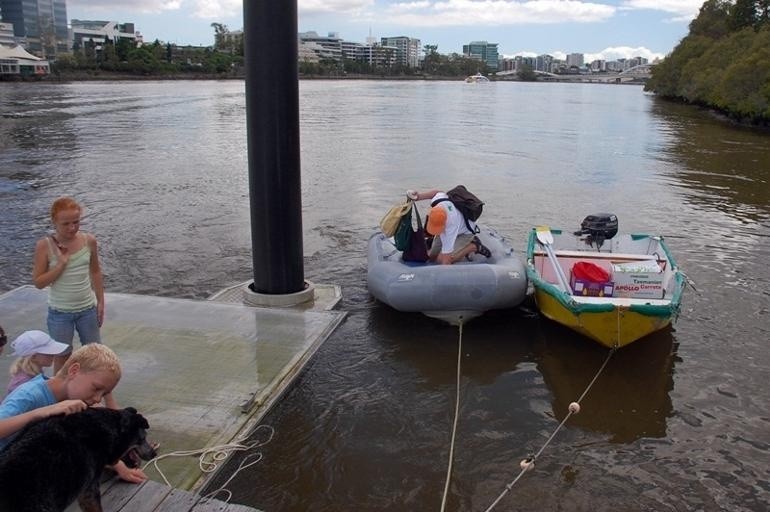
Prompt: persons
<box><xmin>0</xmin><ymin>328</ymin><xmax>147</xmax><ymax>484</ymax></box>
<box><xmin>411</xmin><ymin>190</ymin><xmax>491</xmax><ymax>265</ymax></box>
<box><xmin>33</xmin><ymin>198</ymin><xmax>118</xmax><ymax>410</ymax></box>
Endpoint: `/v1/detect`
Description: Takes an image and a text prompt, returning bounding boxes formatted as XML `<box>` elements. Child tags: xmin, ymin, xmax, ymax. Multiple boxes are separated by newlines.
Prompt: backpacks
<box><xmin>431</xmin><ymin>184</ymin><xmax>485</xmax><ymax>235</ymax></box>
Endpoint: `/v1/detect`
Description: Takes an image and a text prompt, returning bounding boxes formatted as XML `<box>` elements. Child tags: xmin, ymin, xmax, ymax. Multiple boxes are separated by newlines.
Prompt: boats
<box><xmin>466</xmin><ymin>75</ymin><xmax>492</xmax><ymax>85</ymax></box>
<box><xmin>527</xmin><ymin>223</ymin><xmax>691</xmax><ymax>354</ymax></box>
<box><xmin>366</xmin><ymin>205</ymin><xmax>529</xmax><ymax>333</ymax></box>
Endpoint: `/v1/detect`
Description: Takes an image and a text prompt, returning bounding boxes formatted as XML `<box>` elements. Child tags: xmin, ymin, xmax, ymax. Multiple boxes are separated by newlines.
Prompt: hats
<box><xmin>11</xmin><ymin>330</ymin><xmax>70</xmax><ymax>355</ymax></box>
<box><xmin>426</xmin><ymin>206</ymin><xmax>446</xmax><ymax>234</ymax></box>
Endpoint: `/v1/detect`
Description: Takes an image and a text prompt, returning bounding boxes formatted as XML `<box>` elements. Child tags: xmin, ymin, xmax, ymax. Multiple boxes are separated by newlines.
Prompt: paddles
<box><xmin>535</xmin><ymin>225</ymin><xmax>573</xmax><ymax>296</ymax></box>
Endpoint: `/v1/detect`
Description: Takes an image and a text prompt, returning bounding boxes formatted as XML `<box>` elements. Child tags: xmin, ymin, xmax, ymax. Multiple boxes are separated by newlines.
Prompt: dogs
<box><xmin>0</xmin><ymin>406</ymin><xmax>158</xmax><ymax>512</ymax></box>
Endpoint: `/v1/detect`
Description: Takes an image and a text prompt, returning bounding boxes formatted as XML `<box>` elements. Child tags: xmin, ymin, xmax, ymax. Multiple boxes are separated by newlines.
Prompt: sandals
<box><xmin>465</xmin><ymin>236</ymin><xmax>492</xmax><ymax>262</ymax></box>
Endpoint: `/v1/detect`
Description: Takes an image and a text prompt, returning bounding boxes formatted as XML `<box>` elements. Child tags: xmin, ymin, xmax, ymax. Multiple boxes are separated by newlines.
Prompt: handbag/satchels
<box><xmin>381</xmin><ymin>202</ymin><xmax>431</xmax><ymax>263</ymax></box>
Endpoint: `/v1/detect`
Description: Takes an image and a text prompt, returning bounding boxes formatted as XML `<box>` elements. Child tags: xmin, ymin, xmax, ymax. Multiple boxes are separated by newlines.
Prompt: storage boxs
<box><xmin>609</xmin><ymin>260</ymin><xmax>664</xmax><ymax>299</ymax></box>
<box><xmin>570</xmin><ymin>266</ymin><xmax>613</xmax><ymax>297</ymax></box>
